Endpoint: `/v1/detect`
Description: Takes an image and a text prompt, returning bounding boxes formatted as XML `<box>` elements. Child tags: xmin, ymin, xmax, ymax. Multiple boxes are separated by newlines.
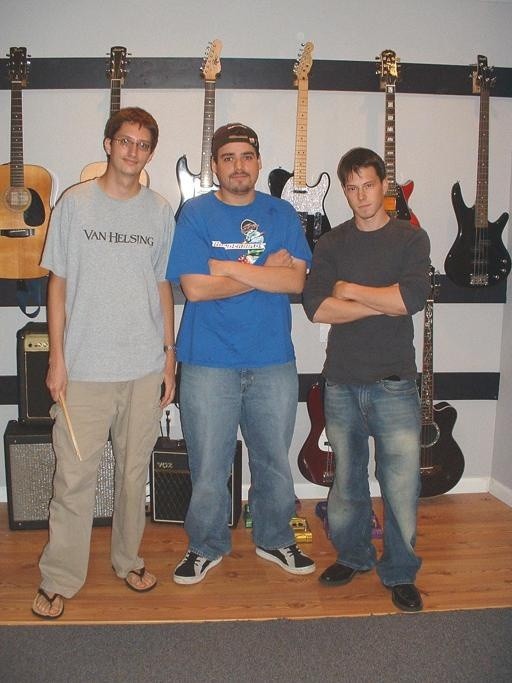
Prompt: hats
<box><xmin>210</xmin><ymin>122</ymin><xmax>260</xmax><ymax>156</ymax></box>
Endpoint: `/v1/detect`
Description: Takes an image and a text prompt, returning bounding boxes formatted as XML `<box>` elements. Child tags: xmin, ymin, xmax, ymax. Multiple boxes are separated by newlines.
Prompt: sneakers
<box><xmin>172</xmin><ymin>549</ymin><xmax>223</xmax><ymax>585</ymax></box>
<box><xmin>254</xmin><ymin>542</ymin><xmax>317</xmax><ymax>576</ymax></box>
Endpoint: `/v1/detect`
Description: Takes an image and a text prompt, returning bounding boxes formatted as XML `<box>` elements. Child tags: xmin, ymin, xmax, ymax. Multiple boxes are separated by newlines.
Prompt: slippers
<box><xmin>31</xmin><ymin>587</ymin><xmax>65</xmax><ymax>620</ymax></box>
<box><xmin>111</xmin><ymin>563</ymin><xmax>158</xmax><ymax>593</ymax></box>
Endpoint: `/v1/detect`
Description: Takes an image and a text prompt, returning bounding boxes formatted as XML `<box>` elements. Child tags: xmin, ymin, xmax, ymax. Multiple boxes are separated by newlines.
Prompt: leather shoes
<box><xmin>318</xmin><ymin>562</ymin><xmax>370</xmax><ymax>587</ymax></box>
<box><xmin>380</xmin><ymin>580</ymin><xmax>424</xmax><ymax>612</ymax></box>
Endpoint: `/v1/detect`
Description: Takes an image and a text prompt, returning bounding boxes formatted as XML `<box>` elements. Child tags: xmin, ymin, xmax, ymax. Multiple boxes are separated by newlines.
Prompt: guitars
<box><xmin>374</xmin><ymin>48</ymin><xmax>422</xmax><ymax>233</ymax></box>
<box><xmin>80</xmin><ymin>46</ymin><xmax>151</xmax><ymax>190</ymax></box>
<box><xmin>173</xmin><ymin>38</ymin><xmax>224</xmax><ymax>225</ymax></box>
<box><xmin>267</xmin><ymin>42</ymin><xmax>334</xmax><ymax>284</ymax></box>
<box><xmin>0</xmin><ymin>45</ymin><xmax>54</xmax><ymax>281</ymax></box>
<box><xmin>419</xmin><ymin>262</ymin><xmax>465</xmax><ymax>497</ymax></box>
<box><xmin>297</xmin><ymin>383</ymin><xmax>337</xmax><ymax>487</ymax></box>
<box><xmin>443</xmin><ymin>53</ymin><xmax>512</xmax><ymax>290</ymax></box>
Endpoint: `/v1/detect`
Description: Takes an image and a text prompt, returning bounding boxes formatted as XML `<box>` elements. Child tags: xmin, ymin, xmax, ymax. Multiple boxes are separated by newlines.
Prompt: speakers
<box><xmin>15</xmin><ymin>330</ymin><xmax>75</xmax><ymax>427</ymax></box>
<box><xmin>151</xmin><ymin>436</ymin><xmax>242</xmax><ymax>527</ymax></box>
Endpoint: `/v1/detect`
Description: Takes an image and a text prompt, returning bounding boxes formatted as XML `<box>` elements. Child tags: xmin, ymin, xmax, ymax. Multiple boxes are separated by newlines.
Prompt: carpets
<box><xmin>1</xmin><ymin>605</ymin><xmax>512</xmax><ymax>681</ymax></box>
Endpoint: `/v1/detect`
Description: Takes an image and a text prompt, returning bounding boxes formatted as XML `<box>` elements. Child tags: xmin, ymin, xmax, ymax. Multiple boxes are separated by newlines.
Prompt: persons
<box><xmin>300</xmin><ymin>148</ymin><xmax>430</xmax><ymax>612</ymax></box>
<box><xmin>30</xmin><ymin>108</ymin><xmax>175</xmax><ymax>618</ymax></box>
<box><xmin>163</xmin><ymin>118</ymin><xmax>312</xmax><ymax>582</ymax></box>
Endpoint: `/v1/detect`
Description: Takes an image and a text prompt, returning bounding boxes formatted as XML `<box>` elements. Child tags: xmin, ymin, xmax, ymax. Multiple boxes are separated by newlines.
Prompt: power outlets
<box><xmin>160</xmin><ymin>405</ymin><xmax>175</xmax><ymax>427</ymax></box>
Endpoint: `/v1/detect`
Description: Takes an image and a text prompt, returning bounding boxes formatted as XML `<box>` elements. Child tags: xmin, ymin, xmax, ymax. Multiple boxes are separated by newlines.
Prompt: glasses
<box><xmin>112</xmin><ymin>136</ymin><xmax>153</xmax><ymax>153</ymax></box>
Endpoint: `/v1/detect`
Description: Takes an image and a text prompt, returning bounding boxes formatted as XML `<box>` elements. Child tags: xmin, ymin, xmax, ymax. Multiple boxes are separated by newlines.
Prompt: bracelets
<box><xmin>163</xmin><ymin>344</ymin><xmax>176</xmax><ymax>352</ymax></box>
